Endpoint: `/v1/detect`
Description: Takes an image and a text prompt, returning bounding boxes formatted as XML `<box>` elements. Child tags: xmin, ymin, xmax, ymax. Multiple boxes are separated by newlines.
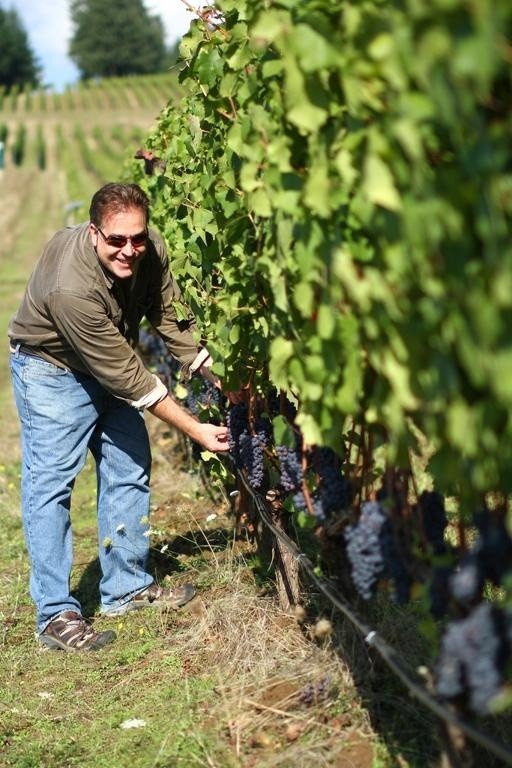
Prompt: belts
<box><xmin>10</xmin><ymin>339</ymin><xmax>38</xmax><ymax>357</ymax></box>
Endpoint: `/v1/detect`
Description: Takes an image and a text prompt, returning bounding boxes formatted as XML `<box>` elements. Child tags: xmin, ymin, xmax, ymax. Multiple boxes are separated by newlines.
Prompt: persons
<box><xmin>7</xmin><ymin>179</ymin><xmax>255</xmax><ymax>652</ymax></box>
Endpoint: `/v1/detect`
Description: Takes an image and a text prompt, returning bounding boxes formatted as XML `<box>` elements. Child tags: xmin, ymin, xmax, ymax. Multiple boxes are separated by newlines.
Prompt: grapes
<box><xmin>137</xmin><ymin>322</ymin><xmax>512</xmax><ymax>720</ymax></box>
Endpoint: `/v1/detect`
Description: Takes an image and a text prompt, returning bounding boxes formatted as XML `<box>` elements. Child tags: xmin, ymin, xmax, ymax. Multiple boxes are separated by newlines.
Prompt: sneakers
<box><xmin>105</xmin><ymin>583</ymin><xmax>196</xmax><ymax>618</ymax></box>
<box><xmin>38</xmin><ymin>610</ymin><xmax>117</xmax><ymax>651</ymax></box>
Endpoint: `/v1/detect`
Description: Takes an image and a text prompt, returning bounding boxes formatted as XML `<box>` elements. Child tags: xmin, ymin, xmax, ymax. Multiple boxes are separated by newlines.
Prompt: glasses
<box><xmin>93</xmin><ymin>222</ymin><xmax>150</xmax><ymax>249</ymax></box>
